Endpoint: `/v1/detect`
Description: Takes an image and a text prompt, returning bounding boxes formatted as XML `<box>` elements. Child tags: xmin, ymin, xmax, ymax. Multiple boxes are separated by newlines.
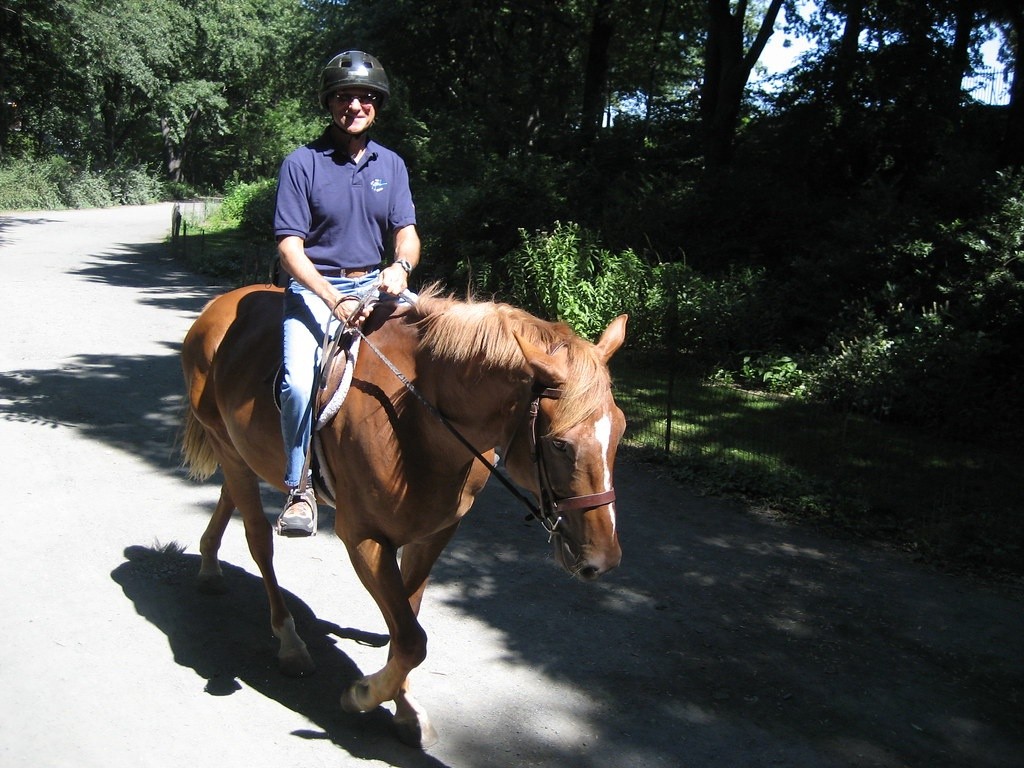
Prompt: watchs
<box><xmin>394</xmin><ymin>259</ymin><xmax>412</xmax><ymax>278</ymax></box>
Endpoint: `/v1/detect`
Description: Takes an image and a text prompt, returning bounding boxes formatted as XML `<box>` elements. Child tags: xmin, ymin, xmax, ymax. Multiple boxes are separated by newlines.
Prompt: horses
<box><xmin>181</xmin><ymin>280</ymin><xmax>628</xmax><ymax>749</ymax></box>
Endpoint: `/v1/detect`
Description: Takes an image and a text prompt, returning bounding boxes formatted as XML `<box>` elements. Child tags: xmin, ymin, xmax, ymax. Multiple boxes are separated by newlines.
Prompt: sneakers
<box><xmin>279</xmin><ymin>487</ymin><xmax>318</xmax><ymax>534</ymax></box>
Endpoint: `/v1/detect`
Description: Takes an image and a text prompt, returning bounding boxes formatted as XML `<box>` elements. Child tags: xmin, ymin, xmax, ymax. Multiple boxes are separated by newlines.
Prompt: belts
<box><xmin>319</xmin><ymin>266</ymin><xmax>373</xmax><ymax>279</ymax></box>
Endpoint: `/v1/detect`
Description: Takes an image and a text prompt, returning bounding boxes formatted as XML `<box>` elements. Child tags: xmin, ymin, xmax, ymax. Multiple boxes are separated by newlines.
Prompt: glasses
<box><xmin>334</xmin><ymin>93</ymin><xmax>379</xmax><ymax>105</ymax></box>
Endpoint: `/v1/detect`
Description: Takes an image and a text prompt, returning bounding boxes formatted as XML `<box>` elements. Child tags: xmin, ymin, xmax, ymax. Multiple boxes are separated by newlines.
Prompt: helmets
<box><xmin>318</xmin><ymin>51</ymin><xmax>391</xmax><ymax>111</ymax></box>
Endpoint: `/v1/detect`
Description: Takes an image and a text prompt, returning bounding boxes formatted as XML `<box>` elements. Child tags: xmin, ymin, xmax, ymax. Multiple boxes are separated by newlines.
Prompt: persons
<box><xmin>273</xmin><ymin>50</ymin><xmax>423</xmax><ymax>538</ymax></box>
